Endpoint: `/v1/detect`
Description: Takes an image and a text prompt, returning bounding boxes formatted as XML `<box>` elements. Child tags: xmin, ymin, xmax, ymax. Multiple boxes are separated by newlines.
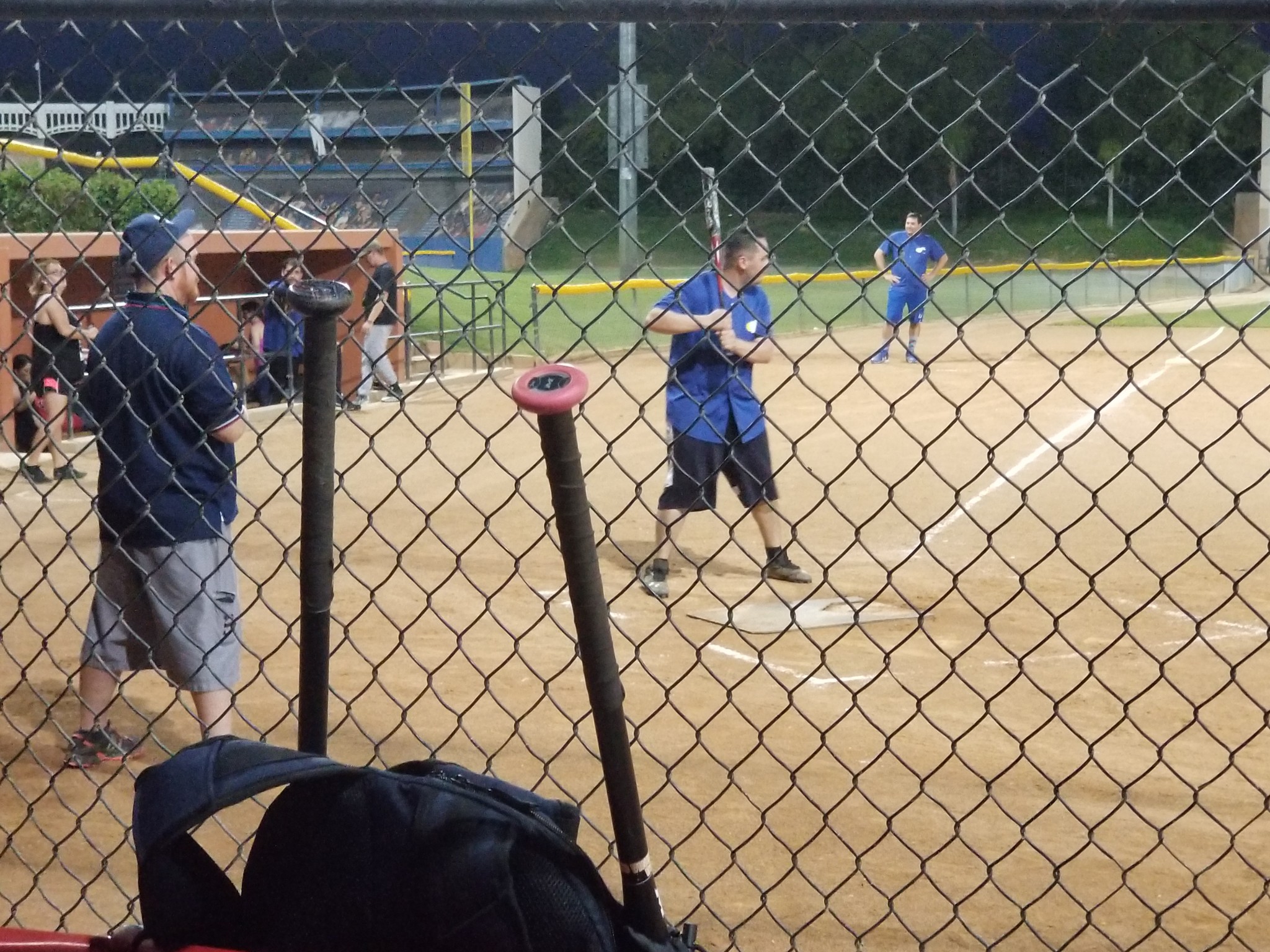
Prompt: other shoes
<box><xmin>346</xmin><ymin>402</ymin><xmax>362</xmax><ymax>411</ymax></box>
<box><xmin>381</xmin><ymin>390</ymin><xmax>405</xmax><ymax>402</ymax></box>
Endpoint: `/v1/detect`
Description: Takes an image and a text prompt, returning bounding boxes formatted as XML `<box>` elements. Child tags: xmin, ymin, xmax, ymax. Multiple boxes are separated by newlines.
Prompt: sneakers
<box><xmin>906</xmin><ymin>350</ymin><xmax>917</xmax><ymax>364</ymax></box>
<box><xmin>870</xmin><ymin>349</ymin><xmax>889</xmax><ymax>364</ymax></box>
<box><xmin>53</xmin><ymin>465</ymin><xmax>87</xmax><ymax>479</ymax></box>
<box><xmin>23</xmin><ymin>466</ymin><xmax>52</xmax><ymax>483</ymax></box>
<box><xmin>64</xmin><ymin>721</ymin><xmax>144</xmax><ymax>767</ymax></box>
<box><xmin>645</xmin><ymin>564</ymin><xmax>669</xmax><ymax>598</ymax></box>
<box><xmin>765</xmin><ymin>551</ymin><xmax>809</xmax><ymax>583</ymax></box>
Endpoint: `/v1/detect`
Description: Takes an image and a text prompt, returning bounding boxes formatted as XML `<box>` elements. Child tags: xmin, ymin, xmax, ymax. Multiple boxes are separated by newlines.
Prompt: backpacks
<box><xmin>130</xmin><ymin>734</ymin><xmax>623</xmax><ymax>952</ymax></box>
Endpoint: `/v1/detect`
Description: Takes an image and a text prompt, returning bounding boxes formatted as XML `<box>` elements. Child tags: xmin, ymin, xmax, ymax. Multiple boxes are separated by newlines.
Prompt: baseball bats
<box><xmin>287</xmin><ymin>278</ymin><xmax>355</xmax><ymax>762</ymax></box>
<box><xmin>512</xmin><ymin>364</ymin><xmax>672</xmax><ymax>939</ymax></box>
<box><xmin>700</xmin><ymin>167</ymin><xmax>735</xmax><ymax>357</ymax></box>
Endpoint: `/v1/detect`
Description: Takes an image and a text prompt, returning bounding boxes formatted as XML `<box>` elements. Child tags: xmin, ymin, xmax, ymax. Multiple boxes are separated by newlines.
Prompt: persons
<box><xmin>12</xmin><ymin>183</ymin><xmax>515</xmax><ymax>483</ymax></box>
<box><xmin>61</xmin><ymin>212</ymin><xmax>246</xmax><ymax>769</ymax></box>
<box><xmin>638</xmin><ymin>225</ymin><xmax>814</xmax><ymax>596</ymax></box>
<box><xmin>868</xmin><ymin>213</ymin><xmax>949</xmax><ymax>365</ymax></box>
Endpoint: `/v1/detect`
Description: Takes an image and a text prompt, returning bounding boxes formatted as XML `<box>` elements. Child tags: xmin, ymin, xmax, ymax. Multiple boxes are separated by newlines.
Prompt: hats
<box><xmin>358</xmin><ymin>239</ymin><xmax>383</xmax><ymax>257</ymax></box>
<box><xmin>120</xmin><ymin>207</ymin><xmax>195</xmax><ymax>277</ymax></box>
